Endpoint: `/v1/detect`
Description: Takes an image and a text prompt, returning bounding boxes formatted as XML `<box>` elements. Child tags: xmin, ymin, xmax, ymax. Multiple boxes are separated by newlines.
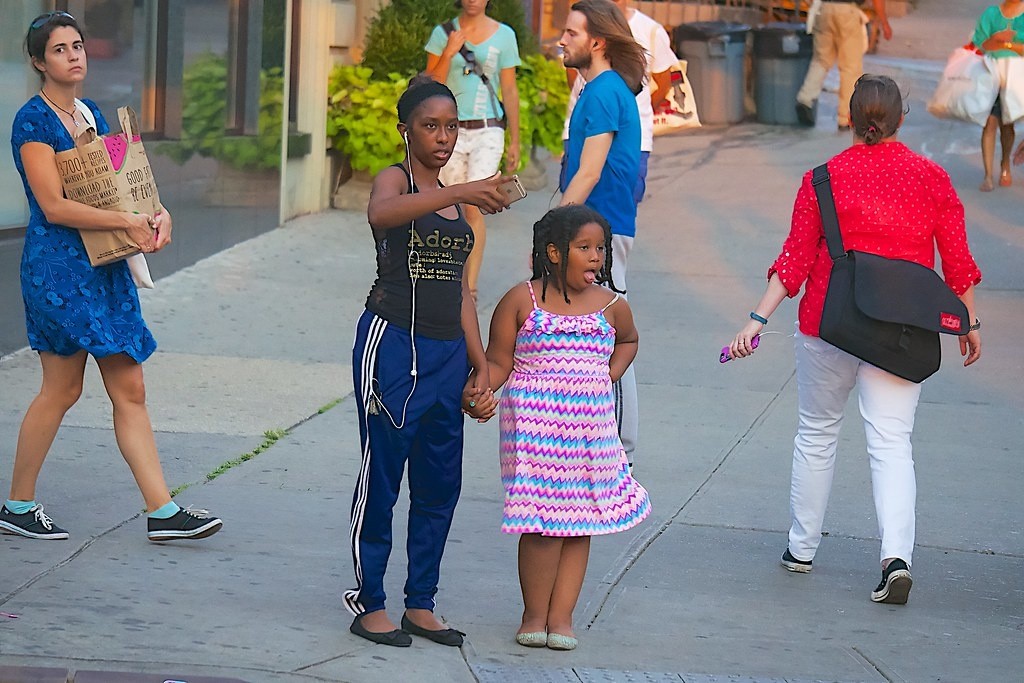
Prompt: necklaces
<box><xmin>41</xmin><ymin>87</ymin><xmax>80</xmax><ymax>127</ymax></box>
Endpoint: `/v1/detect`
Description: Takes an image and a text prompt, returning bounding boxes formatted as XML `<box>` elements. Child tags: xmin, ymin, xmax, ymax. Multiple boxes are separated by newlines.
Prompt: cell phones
<box><xmin>478</xmin><ymin>174</ymin><xmax>528</xmax><ymax>215</ymax></box>
<box><xmin>719</xmin><ymin>333</ymin><xmax>760</xmax><ymax>363</ymax></box>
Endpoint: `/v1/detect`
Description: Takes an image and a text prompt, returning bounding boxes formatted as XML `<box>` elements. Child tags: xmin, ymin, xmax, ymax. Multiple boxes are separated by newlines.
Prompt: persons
<box><xmin>561</xmin><ymin>0</ymin><xmax>679</xmax><ymax>216</ymax></box>
<box><xmin>0</xmin><ymin>11</ymin><xmax>223</xmax><ymax>542</ymax></box>
<box><xmin>461</xmin><ymin>205</ymin><xmax>652</xmax><ymax>650</ymax></box>
<box><xmin>972</xmin><ymin>0</ymin><xmax>1024</xmax><ymax>192</ymax></box>
<box><xmin>795</xmin><ymin>0</ymin><xmax>892</xmax><ymax>131</ymax></box>
<box><xmin>557</xmin><ymin>0</ymin><xmax>648</xmax><ymax>477</ymax></box>
<box><xmin>424</xmin><ymin>0</ymin><xmax>522</xmax><ymax>307</ymax></box>
<box><xmin>340</xmin><ymin>76</ymin><xmax>490</xmax><ymax>647</ymax></box>
<box><xmin>729</xmin><ymin>72</ymin><xmax>982</xmax><ymax>605</ymax></box>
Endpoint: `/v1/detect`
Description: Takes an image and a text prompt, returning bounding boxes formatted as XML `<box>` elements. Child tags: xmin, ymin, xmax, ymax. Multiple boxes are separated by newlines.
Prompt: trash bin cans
<box><xmin>674</xmin><ymin>21</ymin><xmax>752</xmax><ymax>122</ymax></box>
<box><xmin>755</xmin><ymin>22</ymin><xmax>818</xmax><ymax>125</ymax></box>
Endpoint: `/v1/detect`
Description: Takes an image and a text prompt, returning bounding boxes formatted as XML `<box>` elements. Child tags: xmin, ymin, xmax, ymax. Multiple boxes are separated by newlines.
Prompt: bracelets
<box><xmin>1005</xmin><ymin>42</ymin><xmax>1012</xmax><ymax>48</ymax></box>
<box><xmin>750</xmin><ymin>312</ymin><xmax>768</xmax><ymax>324</ymax></box>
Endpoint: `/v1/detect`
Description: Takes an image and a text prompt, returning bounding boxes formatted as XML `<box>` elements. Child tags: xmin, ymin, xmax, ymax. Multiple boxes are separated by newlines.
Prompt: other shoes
<box><xmin>400</xmin><ymin>612</ymin><xmax>464</xmax><ymax>646</ymax></box>
<box><xmin>517</xmin><ymin>625</ymin><xmax>548</xmax><ymax>647</ymax></box>
<box><xmin>999</xmin><ymin>166</ymin><xmax>1011</xmax><ymax>186</ymax></box>
<box><xmin>979</xmin><ymin>178</ymin><xmax>994</xmax><ymax>192</ymax></box>
<box><xmin>796</xmin><ymin>104</ymin><xmax>815</xmax><ymax>127</ymax></box>
<box><xmin>351</xmin><ymin>617</ymin><xmax>412</xmax><ymax>646</ymax></box>
<box><xmin>838</xmin><ymin>125</ymin><xmax>850</xmax><ymax>132</ymax></box>
<box><xmin>547</xmin><ymin>632</ymin><xmax>577</xmax><ymax>650</ymax></box>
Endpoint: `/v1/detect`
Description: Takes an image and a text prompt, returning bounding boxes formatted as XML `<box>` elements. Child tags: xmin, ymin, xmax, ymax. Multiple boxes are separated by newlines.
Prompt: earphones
<box><xmin>592</xmin><ymin>41</ymin><xmax>598</xmax><ymax>48</ymax></box>
<box><xmin>404</xmin><ymin>130</ymin><xmax>409</xmax><ymax>145</ymax></box>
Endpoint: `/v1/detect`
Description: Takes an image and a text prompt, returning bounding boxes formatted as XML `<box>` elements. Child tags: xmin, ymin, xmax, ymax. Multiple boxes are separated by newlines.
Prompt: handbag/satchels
<box><xmin>648</xmin><ymin>22</ymin><xmax>702</xmax><ymax>136</ymax></box>
<box><xmin>926</xmin><ymin>47</ymin><xmax>1000</xmax><ymax>128</ymax></box>
<box><xmin>997</xmin><ymin>55</ymin><xmax>1024</xmax><ymax>125</ymax></box>
<box><xmin>818</xmin><ymin>250</ymin><xmax>969</xmax><ymax>384</ymax></box>
<box><xmin>57</xmin><ymin>106</ymin><xmax>161</xmax><ymax>267</ymax></box>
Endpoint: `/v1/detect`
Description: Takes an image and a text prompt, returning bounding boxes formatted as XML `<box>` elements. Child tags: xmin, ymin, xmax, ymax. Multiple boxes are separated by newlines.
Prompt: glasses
<box><xmin>30</xmin><ymin>10</ymin><xmax>77</xmax><ymax>36</ymax></box>
<box><xmin>462</xmin><ymin>51</ymin><xmax>475</xmax><ymax>75</ymax></box>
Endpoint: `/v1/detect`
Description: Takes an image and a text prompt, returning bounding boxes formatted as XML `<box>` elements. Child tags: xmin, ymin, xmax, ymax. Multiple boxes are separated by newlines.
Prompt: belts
<box><xmin>458</xmin><ymin>119</ymin><xmax>505</xmax><ymax>129</ymax></box>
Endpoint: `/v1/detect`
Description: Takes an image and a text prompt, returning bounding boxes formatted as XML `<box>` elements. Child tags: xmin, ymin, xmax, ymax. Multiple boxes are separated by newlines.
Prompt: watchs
<box><xmin>967</xmin><ymin>317</ymin><xmax>981</xmax><ymax>331</ymax></box>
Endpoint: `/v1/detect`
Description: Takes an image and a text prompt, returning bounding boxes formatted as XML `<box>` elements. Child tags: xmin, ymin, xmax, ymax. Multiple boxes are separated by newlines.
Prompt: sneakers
<box><xmin>780</xmin><ymin>545</ymin><xmax>813</xmax><ymax>573</ymax></box>
<box><xmin>0</xmin><ymin>503</ymin><xmax>69</xmax><ymax>539</ymax></box>
<box><xmin>870</xmin><ymin>558</ymin><xmax>913</xmax><ymax>604</ymax></box>
<box><xmin>147</xmin><ymin>503</ymin><xmax>222</xmax><ymax>540</ymax></box>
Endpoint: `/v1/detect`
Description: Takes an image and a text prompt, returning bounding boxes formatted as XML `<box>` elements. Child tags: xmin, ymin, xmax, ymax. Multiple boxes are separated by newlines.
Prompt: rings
<box><xmin>147</xmin><ymin>244</ymin><xmax>151</xmax><ymax>248</ymax></box>
<box><xmin>148</xmin><ymin>216</ymin><xmax>151</xmax><ymax>222</ymax></box>
<box><xmin>739</xmin><ymin>342</ymin><xmax>744</xmax><ymax>344</ymax></box>
